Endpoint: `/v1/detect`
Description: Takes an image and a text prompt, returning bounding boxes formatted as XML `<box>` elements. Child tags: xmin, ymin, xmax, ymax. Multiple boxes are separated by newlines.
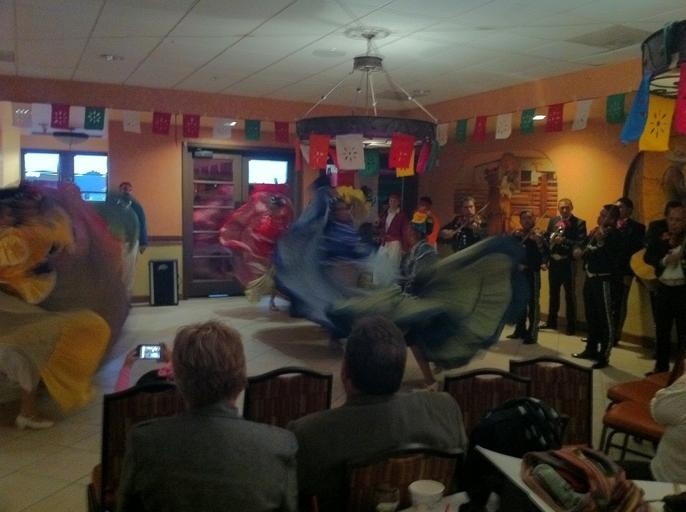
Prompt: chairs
<box><xmin>442</xmin><ymin>366</ymin><xmax>533</xmax><ymax>427</ymax></box>
<box><xmin>343</xmin><ymin>438</ymin><xmax>444</xmax><ymax>512</ymax></box>
<box><xmin>241</xmin><ymin>366</ymin><xmax>335</xmax><ymax>425</ymax></box>
<box><xmin>507</xmin><ymin>355</ymin><xmax>595</xmax><ymax>450</ymax></box>
<box><xmin>86</xmin><ymin>384</ymin><xmax>188</xmax><ymax>510</ymax></box>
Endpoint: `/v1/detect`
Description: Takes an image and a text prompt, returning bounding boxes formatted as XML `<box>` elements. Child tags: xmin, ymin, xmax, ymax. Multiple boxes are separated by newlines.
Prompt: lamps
<box><xmin>296</xmin><ymin>26</ymin><xmax>438</xmax><ymax>150</ymax></box>
<box><xmin>54</xmin><ymin>132</ymin><xmax>87</xmax><ymax>146</ymax></box>
<box><xmin>640</xmin><ymin>18</ymin><xmax>685</xmax><ymax>99</ymax></box>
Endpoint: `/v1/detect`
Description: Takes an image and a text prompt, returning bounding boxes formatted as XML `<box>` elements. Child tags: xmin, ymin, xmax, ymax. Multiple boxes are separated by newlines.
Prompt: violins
<box><xmin>458</xmin><ymin>216</ymin><xmax>482</xmax><ymax>229</ymax></box>
<box><xmin>529</xmin><ymin>228</ymin><xmax>546</xmax><ymax>242</ymax></box>
<box><xmin>595</xmin><ymin>225</ymin><xmax>614</xmax><ymax>240</ymax></box>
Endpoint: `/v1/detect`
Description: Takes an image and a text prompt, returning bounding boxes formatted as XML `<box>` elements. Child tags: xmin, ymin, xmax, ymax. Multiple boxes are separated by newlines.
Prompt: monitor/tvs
<box><xmin>248</xmin><ymin>160</ymin><xmax>288</xmax><ymax>185</ymax></box>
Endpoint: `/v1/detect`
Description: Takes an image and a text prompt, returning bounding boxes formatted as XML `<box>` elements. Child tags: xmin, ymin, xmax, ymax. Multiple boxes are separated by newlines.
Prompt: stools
<box><xmin>597</xmin><ymin>369</ymin><xmax>685</xmax><ymax>474</ymax></box>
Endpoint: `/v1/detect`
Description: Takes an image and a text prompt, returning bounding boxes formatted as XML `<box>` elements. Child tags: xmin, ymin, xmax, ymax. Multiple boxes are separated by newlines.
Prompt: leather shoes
<box><xmin>571</xmin><ymin>353</ymin><xmax>600</xmax><ymax>361</ymax></box>
<box><xmin>592</xmin><ymin>361</ymin><xmax>608</xmax><ymax>369</ymax></box>
<box><xmin>506</xmin><ymin>322</ymin><xmax>576</xmax><ymax>344</ymax></box>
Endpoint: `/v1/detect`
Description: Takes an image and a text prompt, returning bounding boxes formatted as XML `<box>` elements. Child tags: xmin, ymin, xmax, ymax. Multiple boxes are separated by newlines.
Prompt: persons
<box><xmin>115</xmin><ymin>319</ymin><xmax>302</xmax><ymax>512</ymax></box>
<box><xmin>218</xmin><ymin>174</ymin><xmax>684</xmax><ymax>381</ymax></box>
<box><xmin>629</xmin><ymin>358</ymin><xmax>686</xmax><ymax>481</ymax></box>
<box><xmin>284</xmin><ymin>313</ymin><xmax>466</xmax><ymax>512</ymax></box>
<box><xmin>1</xmin><ymin>182</ymin><xmax>147</xmax><ymax>429</ymax></box>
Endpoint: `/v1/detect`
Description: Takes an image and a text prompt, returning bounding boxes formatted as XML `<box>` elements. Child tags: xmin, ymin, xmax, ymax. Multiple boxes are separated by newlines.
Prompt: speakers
<box><xmin>149</xmin><ymin>259</ymin><xmax>178</xmax><ymax>306</ymax></box>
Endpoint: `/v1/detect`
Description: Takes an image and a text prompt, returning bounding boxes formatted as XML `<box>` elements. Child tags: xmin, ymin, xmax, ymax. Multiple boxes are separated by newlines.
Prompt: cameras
<box><xmin>138</xmin><ymin>344</ymin><xmax>165</xmax><ymax>361</ymax></box>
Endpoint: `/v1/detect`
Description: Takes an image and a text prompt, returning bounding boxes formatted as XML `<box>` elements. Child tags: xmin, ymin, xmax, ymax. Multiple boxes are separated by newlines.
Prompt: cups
<box><xmin>407</xmin><ymin>480</ymin><xmax>444</xmax><ymax>505</ymax></box>
<box><xmin>377</xmin><ymin>485</ymin><xmax>399</xmax><ymax>512</ymax></box>
